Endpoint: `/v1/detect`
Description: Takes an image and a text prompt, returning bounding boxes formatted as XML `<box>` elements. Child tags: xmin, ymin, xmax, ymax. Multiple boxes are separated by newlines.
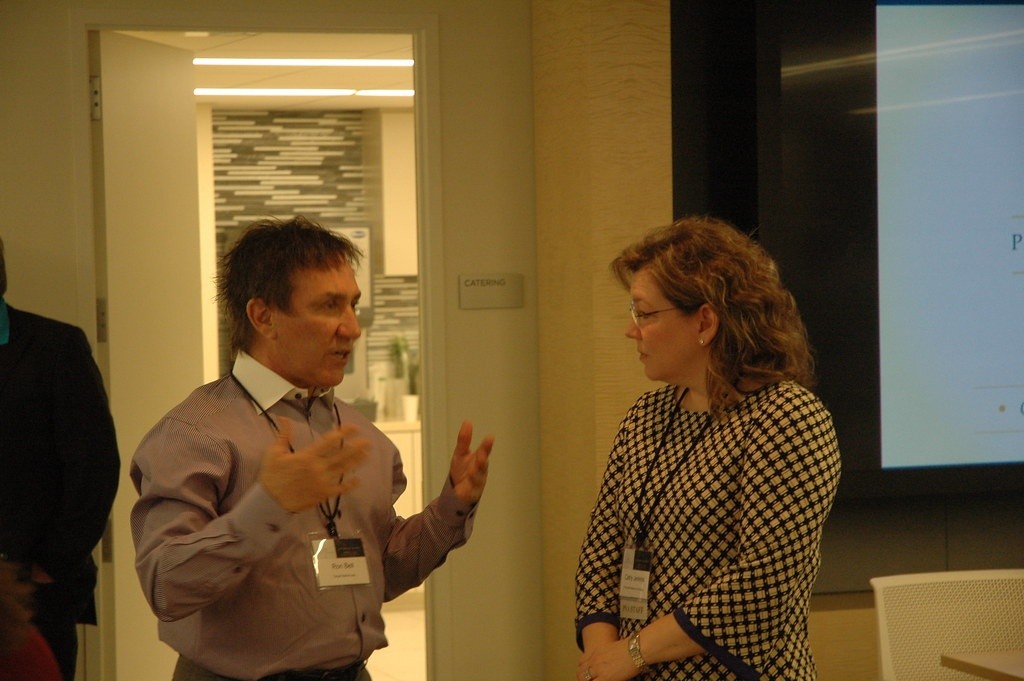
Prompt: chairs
<box><xmin>870</xmin><ymin>568</ymin><xmax>1024</xmax><ymax>681</ymax></box>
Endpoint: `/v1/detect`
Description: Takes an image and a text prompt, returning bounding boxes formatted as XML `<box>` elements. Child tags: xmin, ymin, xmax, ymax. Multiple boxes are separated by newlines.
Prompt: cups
<box><xmin>402</xmin><ymin>394</ymin><xmax>419</xmax><ymax>422</ymax></box>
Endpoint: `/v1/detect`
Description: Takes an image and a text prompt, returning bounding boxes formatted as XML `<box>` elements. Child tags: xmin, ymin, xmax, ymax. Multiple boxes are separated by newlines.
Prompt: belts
<box><xmin>255</xmin><ymin>661</ymin><xmax>364</xmax><ymax>681</ymax></box>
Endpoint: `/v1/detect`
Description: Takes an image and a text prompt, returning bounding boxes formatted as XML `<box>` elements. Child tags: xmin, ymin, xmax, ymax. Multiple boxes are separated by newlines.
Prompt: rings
<box><xmin>583</xmin><ymin>668</ymin><xmax>591</xmax><ymax>681</ymax></box>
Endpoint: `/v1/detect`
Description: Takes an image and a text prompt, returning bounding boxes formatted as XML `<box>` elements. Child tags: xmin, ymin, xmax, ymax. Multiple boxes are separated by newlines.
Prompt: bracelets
<box><xmin>628</xmin><ymin>630</ymin><xmax>652</xmax><ymax>674</ymax></box>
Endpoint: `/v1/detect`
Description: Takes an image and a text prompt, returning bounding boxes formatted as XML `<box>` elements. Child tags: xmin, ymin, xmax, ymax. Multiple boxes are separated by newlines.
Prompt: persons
<box><xmin>0</xmin><ymin>238</ymin><xmax>119</xmax><ymax>681</ymax></box>
<box><xmin>130</xmin><ymin>212</ymin><xmax>494</xmax><ymax>681</ymax></box>
<box><xmin>573</xmin><ymin>215</ymin><xmax>842</xmax><ymax>681</ymax></box>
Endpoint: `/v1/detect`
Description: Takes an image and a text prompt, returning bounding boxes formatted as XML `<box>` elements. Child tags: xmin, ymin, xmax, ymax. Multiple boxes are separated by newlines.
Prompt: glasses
<box><xmin>629</xmin><ymin>301</ymin><xmax>680</xmax><ymax>328</ymax></box>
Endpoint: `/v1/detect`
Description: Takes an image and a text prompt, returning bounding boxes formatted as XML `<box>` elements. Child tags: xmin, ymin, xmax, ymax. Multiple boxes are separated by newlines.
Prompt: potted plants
<box><xmin>400</xmin><ymin>352</ymin><xmax>421</xmax><ymax>423</ymax></box>
